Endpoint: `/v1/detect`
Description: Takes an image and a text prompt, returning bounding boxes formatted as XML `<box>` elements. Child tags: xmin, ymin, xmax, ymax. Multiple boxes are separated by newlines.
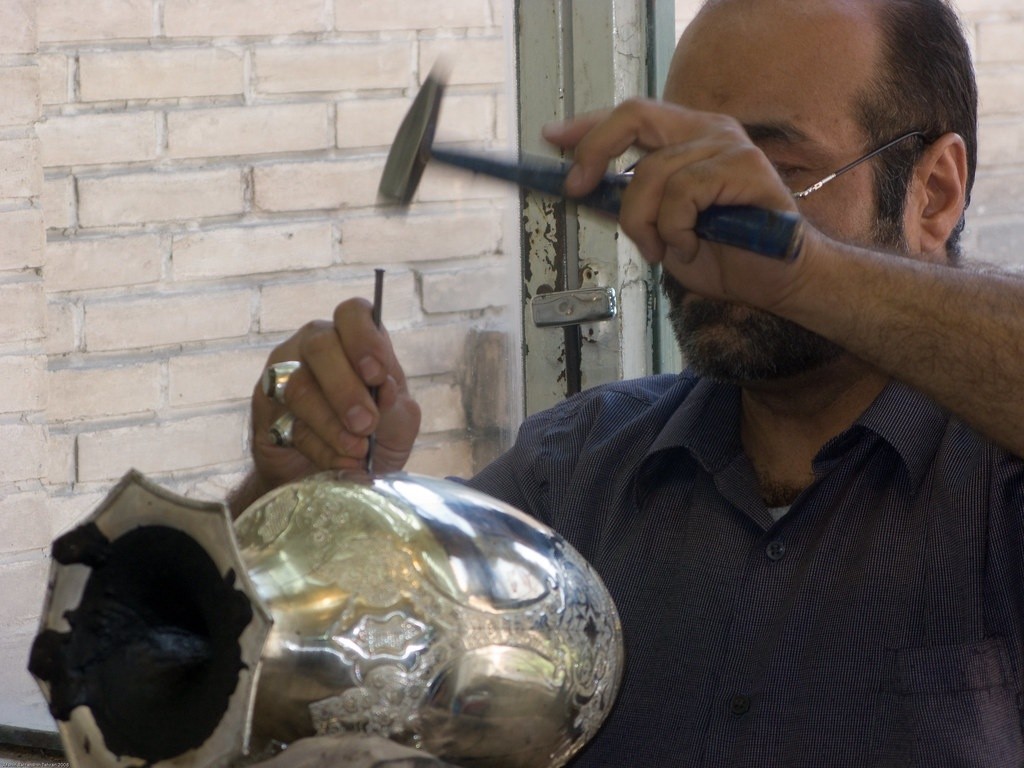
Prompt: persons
<box><xmin>219</xmin><ymin>0</ymin><xmax>1024</xmax><ymax>767</ymax></box>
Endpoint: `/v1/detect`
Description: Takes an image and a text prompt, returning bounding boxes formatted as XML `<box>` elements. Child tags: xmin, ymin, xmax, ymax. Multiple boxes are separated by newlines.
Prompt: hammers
<box><xmin>375</xmin><ymin>73</ymin><xmax>810</xmax><ymax>275</ymax></box>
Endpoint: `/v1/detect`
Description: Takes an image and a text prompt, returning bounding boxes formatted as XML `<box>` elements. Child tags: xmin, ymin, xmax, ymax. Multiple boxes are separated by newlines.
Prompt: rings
<box><xmin>270</xmin><ymin>411</ymin><xmax>294</xmax><ymax>447</ymax></box>
<box><xmin>263</xmin><ymin>361</ymin><xmax>303</xmax><ymax>403</ymax></box>
